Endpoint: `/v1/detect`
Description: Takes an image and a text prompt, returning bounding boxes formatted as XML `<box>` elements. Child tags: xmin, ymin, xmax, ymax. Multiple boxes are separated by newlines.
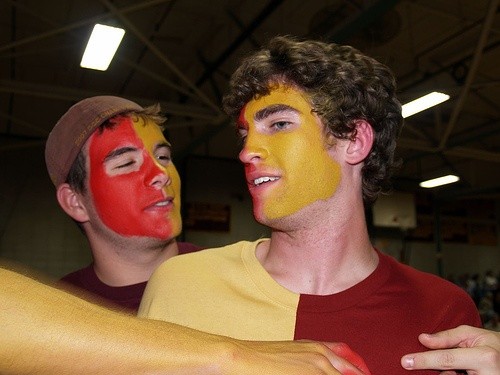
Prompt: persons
<box><xmin>0</xmin><ymin>267</ymin><xmax>371</xmax><ymax>375</ymax></box>
<box><xmin>447</xmin><ymin>270</ymin><xmax>500</xmax><ymax>291</ymax></box>
<box><xmin>134</xmin><ymin>33</ymin><xmax>500</xmax><ymax>375</ymax></box>
<box><xmin>401</xmin><ymin>324</ymin><xmax>499</xmax><ymax>375</ymax></box>
<box><xmin>42</xmin><ymin>93</ymin><xmax>216</xmax><ymax>320</ymax></box>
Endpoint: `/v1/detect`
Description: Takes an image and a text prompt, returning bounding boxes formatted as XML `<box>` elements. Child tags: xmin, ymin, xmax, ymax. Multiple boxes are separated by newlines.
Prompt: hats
<box><xmin>43</xmin><ymin>96</ymin><xmax>147</xmax><ymax>186</ymax></box>
